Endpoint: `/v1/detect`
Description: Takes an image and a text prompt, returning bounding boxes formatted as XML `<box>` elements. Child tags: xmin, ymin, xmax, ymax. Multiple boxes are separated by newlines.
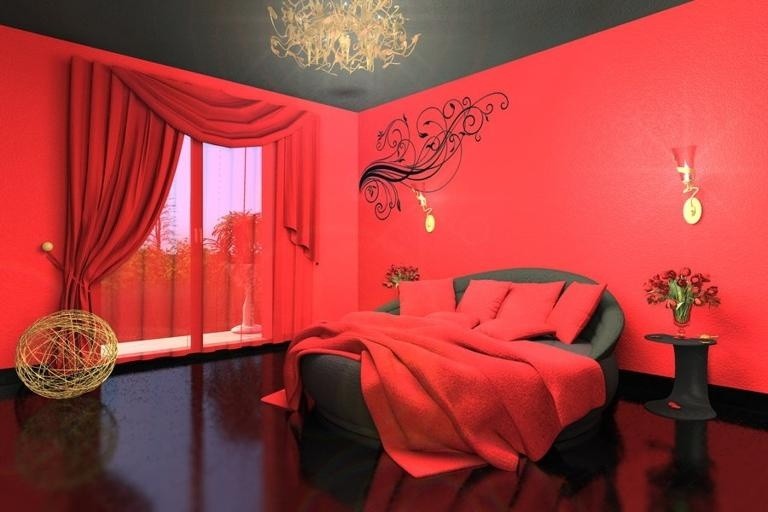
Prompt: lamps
<box><xmin>267</xmin><ymin>0</ymin><xmax>421</xmax><ymax>76</ymax></box>
<box><xmin>411</xmin><ymin>181</ymin><xmax>436</xmax><ymax>232</ymax></box>
<box><xmin>670</xmin><ymin>144</ymin><xmax>703</xmax><ymax>224</ymax></box>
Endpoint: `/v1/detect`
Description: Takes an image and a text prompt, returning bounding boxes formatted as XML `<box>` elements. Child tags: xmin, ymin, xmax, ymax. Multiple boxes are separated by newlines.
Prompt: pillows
<box><xmin>396</xmin><ymin>277</ymin><xmax>608</xmax><ymax>346</ymax></box>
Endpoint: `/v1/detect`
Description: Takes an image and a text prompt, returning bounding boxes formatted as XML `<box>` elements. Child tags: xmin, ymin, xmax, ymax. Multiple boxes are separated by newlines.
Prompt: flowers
<box><xmin>382</xmin><ymin>265</ymin><xmax>421</xmax><ymax>289</ymax></box>
<box><xmin>642</xmin><ymin>267</ymin><xmax>721</xmax><ymax>323</ymax></box>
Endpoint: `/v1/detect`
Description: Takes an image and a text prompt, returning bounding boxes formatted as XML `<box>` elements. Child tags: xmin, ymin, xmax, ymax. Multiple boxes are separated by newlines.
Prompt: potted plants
<box><xmin>202</xmin><ymin>210</ymin><xmax>264</xmax><ymax>334</ymax></box>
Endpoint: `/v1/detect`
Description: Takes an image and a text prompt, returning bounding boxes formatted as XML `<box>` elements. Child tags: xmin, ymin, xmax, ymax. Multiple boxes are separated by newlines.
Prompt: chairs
<box><xmin>260</xmin><ymin>268</ymin><xmax>625</xmax><ymax>479</ymax></box>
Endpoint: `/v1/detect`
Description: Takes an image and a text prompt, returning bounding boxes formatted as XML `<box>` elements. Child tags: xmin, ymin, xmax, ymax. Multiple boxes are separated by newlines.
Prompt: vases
<box><xmin>672</xmin><ymin>302</ymin><xmax>692</xmax><ymax>340</ymax></box>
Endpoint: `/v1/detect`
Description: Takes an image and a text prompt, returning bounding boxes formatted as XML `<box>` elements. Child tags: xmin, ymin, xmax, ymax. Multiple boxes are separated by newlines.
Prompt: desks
<box><xmin>643</xmin><ymin>333</ymin><xmax>717</xmax><ymax>422</ymax></box>
